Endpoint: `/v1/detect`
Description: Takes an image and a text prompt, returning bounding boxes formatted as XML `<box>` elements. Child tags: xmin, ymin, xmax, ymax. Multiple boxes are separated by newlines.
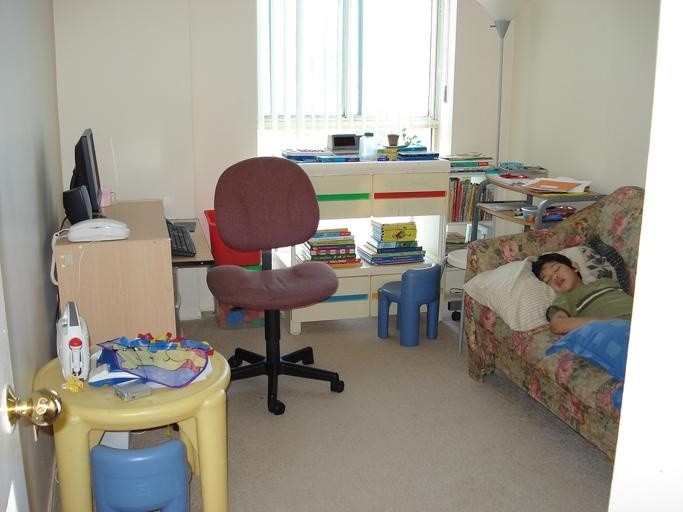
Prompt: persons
<box><xmin>530</xmin><ymin>253</ymin><xmax>634</xmax><ymax>335</ymax></box>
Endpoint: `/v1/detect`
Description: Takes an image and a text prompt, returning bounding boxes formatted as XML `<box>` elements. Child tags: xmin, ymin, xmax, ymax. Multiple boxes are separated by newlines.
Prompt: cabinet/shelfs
<box><xmin>444</xmin><ymin>172</ymin><xmax>607</xmax><ymax>329</ymax></box>
<box><xmin>271</xmin><ymin>159</ymin><xmax>448</xmax><ymax>334</ymax></box>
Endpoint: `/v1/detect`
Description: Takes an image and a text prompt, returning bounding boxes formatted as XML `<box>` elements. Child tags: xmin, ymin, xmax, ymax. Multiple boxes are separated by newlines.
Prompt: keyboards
<box><xmin>167</xmin><ymin>223</ymin><xmax>196</xmax><ymax>257</ymax></box>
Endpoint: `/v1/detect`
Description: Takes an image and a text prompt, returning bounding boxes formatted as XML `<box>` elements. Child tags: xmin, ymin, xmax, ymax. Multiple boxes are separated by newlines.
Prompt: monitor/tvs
<box><xmin>70</xmin><ymin>129</ymin><xmax>106</xmax><ymax>218</ymax></box>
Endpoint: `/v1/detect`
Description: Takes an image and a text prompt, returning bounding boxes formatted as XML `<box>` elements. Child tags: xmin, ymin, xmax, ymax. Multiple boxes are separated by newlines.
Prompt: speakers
<box><xmin>63</xmin><ymin>185</ymin><xmax>93</xmax><ymax>226</ymax></box>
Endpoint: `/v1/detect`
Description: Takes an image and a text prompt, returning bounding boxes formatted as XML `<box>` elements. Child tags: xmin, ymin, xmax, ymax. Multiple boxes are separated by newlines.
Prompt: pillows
<box><xmin>463</xmin><ymin>242</ymin><xmax>617</xmax><ymax>332</ymax></box>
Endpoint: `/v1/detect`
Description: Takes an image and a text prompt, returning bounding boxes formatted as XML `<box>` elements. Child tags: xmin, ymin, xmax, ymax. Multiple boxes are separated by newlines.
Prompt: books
<box><xmin>281</xmin><ymin>142</ymin><xmax>496</xmax><ymax>269</ymax></box>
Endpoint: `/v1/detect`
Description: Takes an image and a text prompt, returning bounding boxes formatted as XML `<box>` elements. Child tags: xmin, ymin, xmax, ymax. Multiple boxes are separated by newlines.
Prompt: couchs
<box><xmin>465</xmin><ymin>187</ymin><xmax>647</xmax><ymax>465</ymax></box>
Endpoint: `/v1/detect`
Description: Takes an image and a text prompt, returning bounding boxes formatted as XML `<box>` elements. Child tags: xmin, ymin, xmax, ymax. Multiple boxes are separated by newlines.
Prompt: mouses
<box><xmin>166</xmin><ymin>219</ymin><xmax>175</xmax><ymax>226</ymax></box>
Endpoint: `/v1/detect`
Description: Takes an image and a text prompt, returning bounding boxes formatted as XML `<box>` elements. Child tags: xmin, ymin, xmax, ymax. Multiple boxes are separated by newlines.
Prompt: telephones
<box><xmin>68</xmin><ymin>218</ymin><xmax>129</xmax><ymax>242</ymax></box>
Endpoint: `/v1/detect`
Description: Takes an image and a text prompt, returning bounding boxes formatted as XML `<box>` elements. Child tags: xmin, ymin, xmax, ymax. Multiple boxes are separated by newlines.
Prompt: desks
<box><xmin>54</xmin><ymin>199</ymin><xmax>214</xmax><ymax>357</ymax></box>
<box><xmin>33</xmin><ymin>346</ymin><xmax>232</xmax><ymax>512</ymax></box>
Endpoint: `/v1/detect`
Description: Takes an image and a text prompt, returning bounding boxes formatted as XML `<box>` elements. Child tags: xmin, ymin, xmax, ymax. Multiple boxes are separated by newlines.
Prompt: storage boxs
<box><xmin>204</xmin><ymin>211</ymin><xmax>265</xmax><ymax>329</ymax></box>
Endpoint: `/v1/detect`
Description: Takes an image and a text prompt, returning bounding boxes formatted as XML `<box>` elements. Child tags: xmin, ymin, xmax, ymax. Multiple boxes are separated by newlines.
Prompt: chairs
<box><xmin>207</xmin><ymin>156</ymin><xmax>345</xmax><ymax>415</ymax></box>
<box><xmin>378</xmin><ymin>262</ymin><xmax>441</xmax><ymax>346</ymax></box>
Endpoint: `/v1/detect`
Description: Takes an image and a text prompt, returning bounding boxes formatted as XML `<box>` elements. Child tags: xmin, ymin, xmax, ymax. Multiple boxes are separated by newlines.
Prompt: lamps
<box><xmin>479</xmin><ymin>0</ymin><xmax>522</xmax><ymax>167</ymax></box>
